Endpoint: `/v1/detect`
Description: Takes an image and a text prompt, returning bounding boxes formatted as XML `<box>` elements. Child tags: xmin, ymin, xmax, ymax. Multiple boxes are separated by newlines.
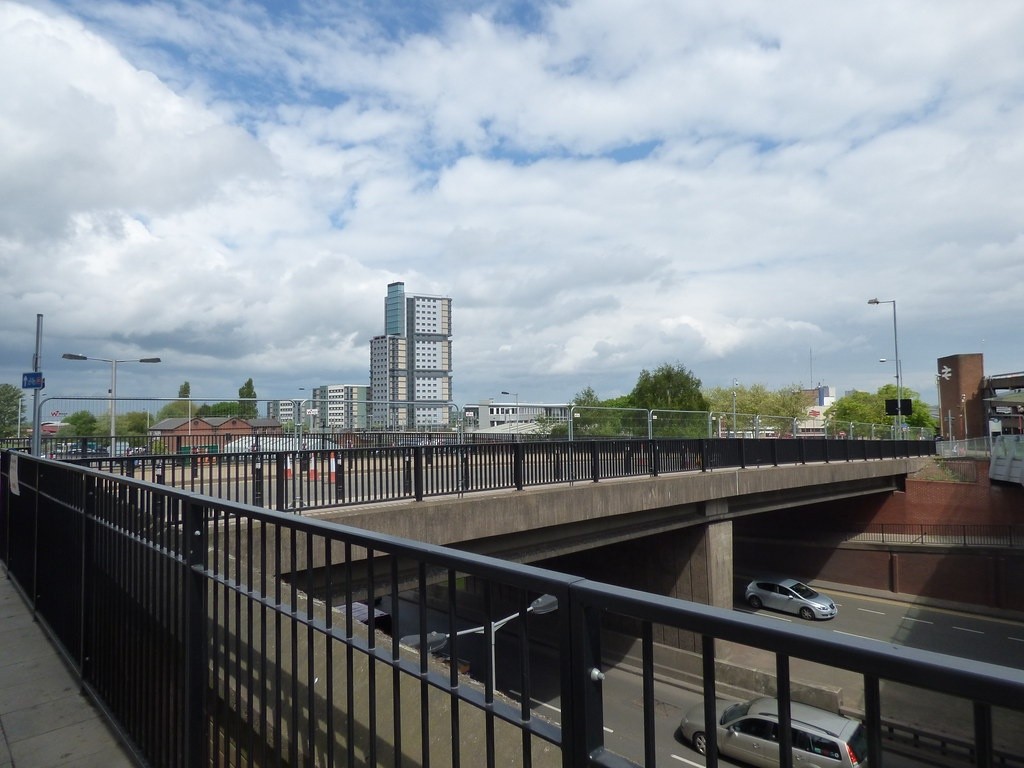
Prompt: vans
<box><xmin>333</xmin><ymin>601</ymin><xmax>392</xmax><ymax>637</ymax></box>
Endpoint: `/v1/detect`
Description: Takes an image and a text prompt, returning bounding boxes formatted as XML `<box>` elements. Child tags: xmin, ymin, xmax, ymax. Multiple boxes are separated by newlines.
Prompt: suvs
<box><xmin>744</xmin><ymin>576</ymin><xmax>838</xmax><ymax>621</ymax></box>
<box><xmin>680</xmin><ymin>696</ymin><xmax>869</xmax><ymax>768</ymax></box>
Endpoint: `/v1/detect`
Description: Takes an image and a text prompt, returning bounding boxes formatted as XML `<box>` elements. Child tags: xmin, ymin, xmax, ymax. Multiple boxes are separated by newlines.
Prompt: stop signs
<box><xmin>839</xmin><ymin>431</ymin><xmax>845</xmax><ymax>436</ymax></box>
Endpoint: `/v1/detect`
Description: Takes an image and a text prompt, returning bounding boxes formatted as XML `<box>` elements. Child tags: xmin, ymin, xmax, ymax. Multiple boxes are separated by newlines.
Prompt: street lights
<box><xmin>868</xmin><ymin>299</ymin><xmax>901</xmax><ymax>440</ymax></box>
<box><xmin>501</xmin><ymin>391</ymin><xmax>519</xmax><ymax>442</ymax></box>
<box><xmin>62</xmin><ymin>353</ymin><xmax>163</xmax><ymax>467</ymax></box>
<box><xmin>878</xmin><ymin>358</ymin><xmax>903</xmax><ymax>401</ymax></box>
<box><xmin>400</xmin><ymin>593</ymin><xmax>559</xmax><ymax>691</ymax></box>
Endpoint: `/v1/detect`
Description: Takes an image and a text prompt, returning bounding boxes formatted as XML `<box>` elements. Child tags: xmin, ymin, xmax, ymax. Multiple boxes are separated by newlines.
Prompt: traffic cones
<box><xmin>325</xmin><ymin>451</ymin><xmax>336</xmax><ymax>484</ymax></box>
<box><xmin>284</xmin><ymin>453</ymin><xmax>298</xmax><ymax>480</ymax></box>
<box><xmin>309</xmin><ymin>452</ymin><xmax>321</xmax><ymax>482</ymax></box>
